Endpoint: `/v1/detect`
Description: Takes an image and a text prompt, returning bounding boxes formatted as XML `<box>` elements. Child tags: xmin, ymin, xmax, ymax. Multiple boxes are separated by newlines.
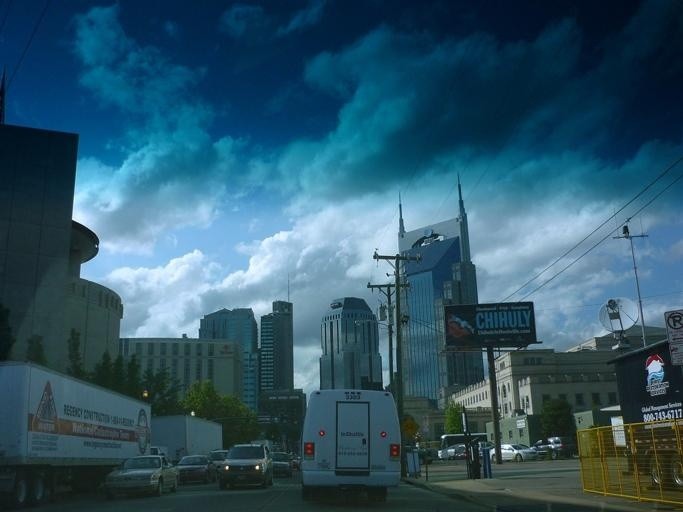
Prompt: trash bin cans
<box><xmin>406</xmin><ymin>447</ymin><xmax>420</xmax><ymax>477</ymax></box>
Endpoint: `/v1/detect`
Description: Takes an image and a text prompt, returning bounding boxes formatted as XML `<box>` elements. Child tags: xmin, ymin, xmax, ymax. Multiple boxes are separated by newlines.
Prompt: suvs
<box><xmin>220</xmin><ymin>442</ymin><xmax>274</xmax><ymax>489</ymax></box>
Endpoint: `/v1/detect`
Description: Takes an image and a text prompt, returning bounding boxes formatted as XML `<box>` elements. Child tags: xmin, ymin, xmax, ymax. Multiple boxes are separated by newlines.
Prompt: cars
<box><xmin>534</xmin><ymin>436</ymin><xmax>574</xmax><ymax>460</ymax></box>
<box><xmin>175</xmin><ymin>454</ymin><xmax>219</xmax><ymax>483</ymax></box>
<box><xmin>104</xmin><ymin>454</ymin><xmax>180</xmax><ymax>496</ymax></box>
<box><xmin>269</xmin><ymin>450</ymin><xmax>293</xmax><ymax>479</ymax></box>
<box><xmin>490</xmin><ymin>444</ymin><xmax>538</xmax><ymax>463</ymax></box>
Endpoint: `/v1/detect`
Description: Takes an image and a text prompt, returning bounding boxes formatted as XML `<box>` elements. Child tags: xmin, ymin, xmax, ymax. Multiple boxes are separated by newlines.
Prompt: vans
<box><xmin>297</xmin><ymin>387</ymin><xmax>403</xmax><ymax>503</ymax></box>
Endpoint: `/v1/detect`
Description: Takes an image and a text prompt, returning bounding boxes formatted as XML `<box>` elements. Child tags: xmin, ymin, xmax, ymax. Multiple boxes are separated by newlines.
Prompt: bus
<box><xmin>437</xmin><ymin>432</ymin><xmax>489</xmax><ymax>462</ymax></box>
